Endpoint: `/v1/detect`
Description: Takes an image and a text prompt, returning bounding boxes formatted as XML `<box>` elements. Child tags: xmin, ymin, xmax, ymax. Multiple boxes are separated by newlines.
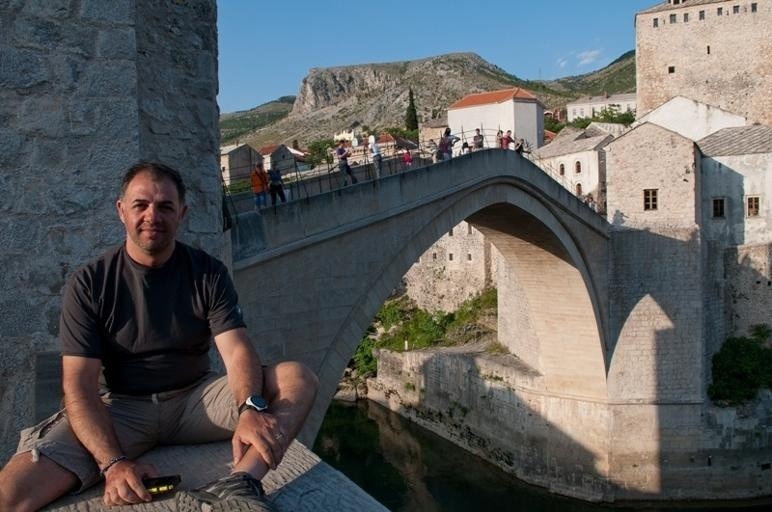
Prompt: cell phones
<box><xmin>141</xmin><ymin>475</ymin><xmax>180</xmax><ymax>494</ymax></box>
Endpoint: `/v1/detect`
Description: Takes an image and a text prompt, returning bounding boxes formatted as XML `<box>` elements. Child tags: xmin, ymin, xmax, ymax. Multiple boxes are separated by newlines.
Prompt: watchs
<box><xmin>238</xmin><ymin>393</ymin><xmax>270</xmax><ymax>415</ymax></box>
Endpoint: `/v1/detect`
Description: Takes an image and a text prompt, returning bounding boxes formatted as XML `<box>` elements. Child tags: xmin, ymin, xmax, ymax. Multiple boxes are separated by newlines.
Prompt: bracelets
<box><xmin>99</xmin><ymin>455</ymin><xmax>129</xmax><ymax>476</ymax></box>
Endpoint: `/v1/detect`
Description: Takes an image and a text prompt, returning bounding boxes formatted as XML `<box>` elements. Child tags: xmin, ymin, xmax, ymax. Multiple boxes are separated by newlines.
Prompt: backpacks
<box><xmin>439</xmin><ymin>137</ymin><xmax>452</xmax><ymax>153</ymax></box>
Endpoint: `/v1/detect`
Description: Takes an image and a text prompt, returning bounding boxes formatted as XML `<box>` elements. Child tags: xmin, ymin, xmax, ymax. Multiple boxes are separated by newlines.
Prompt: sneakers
<box><xmin>174</xmin><ymin>474</ymin><xmax>279</xmax><ymax>512</ymax></box>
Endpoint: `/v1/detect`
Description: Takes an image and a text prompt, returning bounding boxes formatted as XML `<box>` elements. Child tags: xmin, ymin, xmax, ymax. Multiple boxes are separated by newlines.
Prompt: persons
<box><xmin>432</xmin><ymin>128</ymin><xmax>451</xmax><ymax>165</ymax></box>
<box><xmin>336</xmin><ymin>139</ymin><xmax>358</xmax><ymax>187</ymax></box>
<box><xmin>444</xmin><ymin>127</ymin><xmax>461</xmax><ymax>160</ymax></box>
<box><xmin>581</xmin><ymin>195</ymin><xmax>593</xmax><ymax>205</ymax></box>
<box><xmin>266</xmin><ymin>161</ymin><xmax>286</xmax><ymax>205</ymax></box>
<box><xmin>495</xmin><ymin>129</ymin><xmax>504</xmax><ymax>149</ymax></box>
<box><xmin>366</xmin><ymin>134</ymin><xmax>384</xmax><ymax>179</ymax></box>
<box><xmin>425</xmin><ymin>138</ymin><xmax>438</xmax><ymax>154</ymax></box>
<box><xmin>0</xmin><ymin>158</ymin><xmax>320</xmax><ymax>511</ymax></box>
<box><xmin>249</xmin><ymin>160</ymin><xmax>272</xmax><ymax>209</ymax></box>
<box><xmin>515</xmin><ymin>138</ymin><xmax>531</xmax><ymax>156</ymax></box>
<box><xmin>502</xmin><ymin>129</ymin><xmax>512</xmax><ymax>150</ymax></box>
<box><xmin>402</xmin><ymin>147</ymin><xmax>411</xmax><ymax>168</ymax></box>
<box><xmin>473</xmin><ymin>128</ymin><xmax>485</xmax><ymax>152</ymax></box>
